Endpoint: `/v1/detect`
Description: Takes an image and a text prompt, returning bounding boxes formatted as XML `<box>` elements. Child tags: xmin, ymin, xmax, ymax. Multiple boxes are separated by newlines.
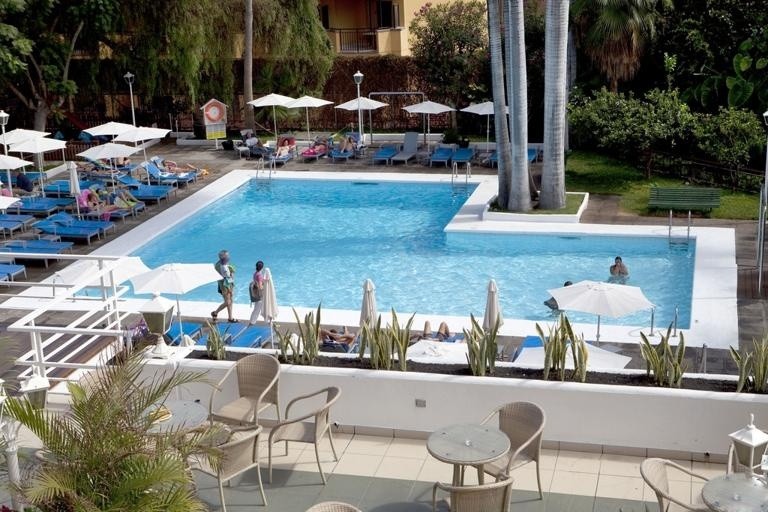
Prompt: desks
<box><xmin>131</xmin><ymin>400</ymin><xmax>207</xmax><ymax>491</ymax></box>
<box><xmin>702</xmin><ymin>472</ymin><xmax>768</xmax><ymax>512</ymax></box>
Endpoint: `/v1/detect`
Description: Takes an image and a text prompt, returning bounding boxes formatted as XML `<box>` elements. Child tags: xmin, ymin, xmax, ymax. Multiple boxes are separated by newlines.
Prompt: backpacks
<box><xmin>248</xmin><ymin>274</ymin><xmax>265</xmax><ymax>302</ymax></box>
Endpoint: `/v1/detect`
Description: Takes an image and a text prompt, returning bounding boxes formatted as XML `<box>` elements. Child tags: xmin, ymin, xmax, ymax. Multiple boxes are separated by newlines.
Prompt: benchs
<box><xmin>648</xmin><ymin>186</ymin><xmax>720</xmax><ymax>218</ymax></box>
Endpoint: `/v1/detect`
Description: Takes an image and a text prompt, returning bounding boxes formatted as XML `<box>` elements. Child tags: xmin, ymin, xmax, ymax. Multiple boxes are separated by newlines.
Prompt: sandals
<box><xmin>228</xmin><ymin>317</ymin><xmax>241</xmax><ymax>323</ymax></box>
<box><xmin>211</xmin><ymin>311</ymin><xmax>217</xmax><ymax>323</ymax></box>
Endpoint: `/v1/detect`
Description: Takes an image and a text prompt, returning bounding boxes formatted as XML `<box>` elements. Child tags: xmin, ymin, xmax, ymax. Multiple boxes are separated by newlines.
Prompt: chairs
<box><xmin>432</xmin><ymin>401</ymin><xmax>546</xmax><ymax>512</ymax></box>
<box><xmin>163</xmin><ymin>321</ymin><xmax>278</xmax><ymax>349</ymax></box>
<box><xmin>34</xmin><ymin>449</ymin><xmax>82</xmax><ymax>468</ymax></box>
<box><xmin>0</xmin><ymin>155</ymin><xmax>202</xmax><ymax>281</ymax></box>
<box><xmin>431</xmin><ymin>331</ymin><xmax>464</xmax><ymax>343</ymax></box>
<box><xmin>640</xmin><ymin>457</ymin><xmax>709</xmax><ymax>512</ymax></box>
<box><xmin>171</xmin><ymin>354</ymin><xmax>364</xmax><ymax>512</ymax></box>
<box><xmin>319</xmin><ymin>331</ymin><xmax>361</xmax><ymax>353</ymax></box>
<box><xmin>514</xmin><ymin>335</ymin><xmax>593</xmax><ymax>359</ymax></box>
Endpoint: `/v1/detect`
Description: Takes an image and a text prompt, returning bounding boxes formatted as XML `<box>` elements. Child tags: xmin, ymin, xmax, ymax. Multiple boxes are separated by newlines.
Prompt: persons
<box><xmin>245</xmin><ymin>131</ymin><xmax>357</xmax><ymax>158</ymax></box>
<box><xmin>248</xmin><ymin>260</ymin><xmax>270</xmax><ymax>327</ymax></box>
<box><xmin>161</xmin><ymin>160</ymin><xmax>199</xmax><ymax>173</ymax></box>
<box><xmin>543</xmin><ymin>281</ymin><xmax>573</xmax><ymax>314</ymax></box>
<box><xmin>610</xmin><ymin>256</ymin><xmax>628</xmax><ymax>275</ymax></box>
<box><xmin>321</xmin><ymin>325</ymin><xmax>362</xmax><ymax>345</ymax></box>
<box><xmin>211</xmin><ymin>250</ymin><xmax>241</xmax><ymax>322</ymax></box>
<box><xmin>410</xmin><ymin>320</ymin><xmax>453</xmax><ymax>342</ymax></box>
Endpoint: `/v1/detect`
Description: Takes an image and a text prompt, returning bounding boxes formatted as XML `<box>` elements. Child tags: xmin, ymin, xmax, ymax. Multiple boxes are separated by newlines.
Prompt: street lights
<box><xmin>0</xmin><ymin>110</ymin><xmax>14</xmax><ymax>198</ymax></box>
<box><xmin>123</xmin><ymin>70</ymin><xmax>138</xmax><ymax>156</ymax></box>
<box><xmin>352</xmin><ymin>70</ymin><xmax>365</xmax><ymax>144</ymax></box>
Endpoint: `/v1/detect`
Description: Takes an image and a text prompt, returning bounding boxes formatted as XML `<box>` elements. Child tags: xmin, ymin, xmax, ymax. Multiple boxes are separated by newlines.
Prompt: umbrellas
<box><xmin>0</xmin><ymin>121</ymin><xmax>172</xmax><ymax>221</ymax></box>
<box><xmin>54</xmin><ymin>254</ymin><xmax>153</xmax><ymax>323</ymax></box>
<box><xmin>129</xmin><ymin>262</ymin><xmax>224</xmax><ymax>340</ymax></box>
<box><xmin>545</xmin><ymin>279</ymin><xmax>656</xmax><ymax>347</ymax></box>
<box><xmin>245</xmin><ymin>92</ymin><xmax>510</xmax><ymax>153</ymax></box>
<box><xmin>359</xmin><ymin>278</ymin><xmax>377</xmax><ymax>349</ymax></box>
<box><xmin>482</xmin><ymin>279</ymin><xmax>505</xmax><ymax>343</ymax></box>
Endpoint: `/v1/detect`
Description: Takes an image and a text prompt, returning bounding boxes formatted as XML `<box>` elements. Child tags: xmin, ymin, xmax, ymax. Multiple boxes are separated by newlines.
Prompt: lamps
<box><xmin>138</xmin><ymin>288</ymin><xmax>177</xmax><ymax>355</ymax></box>
<box><xmin>18</xmin><ymin>365</ymin><xmax>49</xmax><ymax>413</ymax></box>
<box><xmin>728</xmin><ymin>414</ymin><xmax>768</xmax><ymax>476</ymax></box>
